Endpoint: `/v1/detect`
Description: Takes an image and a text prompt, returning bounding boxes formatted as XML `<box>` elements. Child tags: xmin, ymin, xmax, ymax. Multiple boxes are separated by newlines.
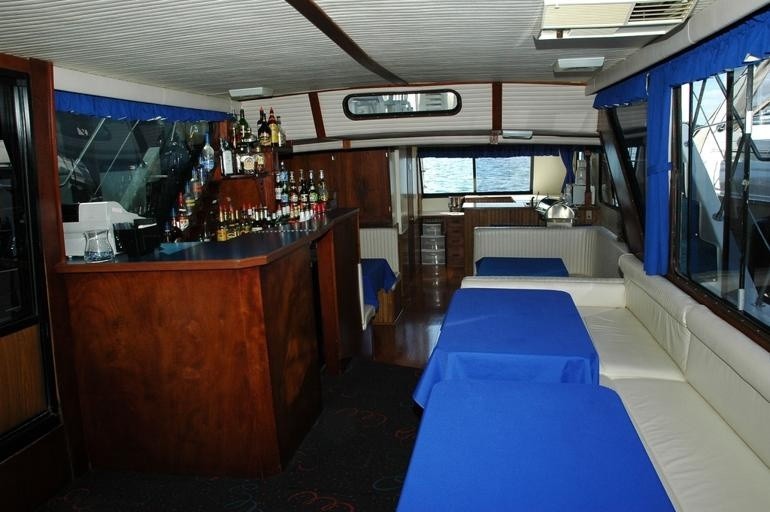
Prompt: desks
<box><xmin>396</xmin><ymin>376</ymin><xmax>676</xmax><ymax>510</ymax></box>
<box><xmin>421</xmin><ymin>288</ymin><xmax>601</xmax><ymax>379</ymax></box>
<box><xmin>474</xmin><ymin>256</ymin><xmax>570</xmax><ymax>277</ymax></box>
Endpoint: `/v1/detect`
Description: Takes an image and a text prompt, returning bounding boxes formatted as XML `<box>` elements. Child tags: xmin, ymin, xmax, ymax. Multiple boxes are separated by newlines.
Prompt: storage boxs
<box><xmin>421</xmin><ymin>222</ymin><xmax>447</xmax><ymax>268</ymax></box>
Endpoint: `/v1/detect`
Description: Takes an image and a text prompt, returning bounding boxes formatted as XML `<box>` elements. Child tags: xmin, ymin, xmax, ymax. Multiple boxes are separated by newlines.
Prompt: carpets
<box><xmin>0</xmin><ymin>350</ymin><xmax>428</xmax><ymax>512</ymax></box>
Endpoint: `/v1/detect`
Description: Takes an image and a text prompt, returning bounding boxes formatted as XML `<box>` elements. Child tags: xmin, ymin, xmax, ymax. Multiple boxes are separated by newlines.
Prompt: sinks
<box><xmin>524</xmin><ymin>202</ymin><xmax>538</xmax><ymax>207</ymax></box>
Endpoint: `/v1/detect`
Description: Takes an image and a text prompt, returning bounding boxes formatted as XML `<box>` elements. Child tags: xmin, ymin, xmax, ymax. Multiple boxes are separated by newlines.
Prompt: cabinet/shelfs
<box><xmin>213</xmin><ymin>141</ymin><xmax>294</xmax><ymax>222</ymax></box>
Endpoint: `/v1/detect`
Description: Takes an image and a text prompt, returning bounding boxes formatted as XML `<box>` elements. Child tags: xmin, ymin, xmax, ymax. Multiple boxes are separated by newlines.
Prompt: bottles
<box><xmin>218</xmin><ymin>106</ymin><xmax>286</xmax><ymax>175</ymax></box>
<box><xmin>274</xmin><ymin>169</ymin><xmax>328</xmax><ymax>221</ymax></box>
<box><xmin>163</xmin><ymin>132</ymin><xmax>215</xmax><ymax>243</ymax></box>
<box><xmin>217</xmin><ymin>204</ymin><xmax>268</xmax><ymax>241</ymax></box>
<box><xmin>449</xmin><ymin>197</ymin><xmax>460</xmax><ymax>212</ymax></box>
<box><xmin>84</xmin><ymin>229</ymin><xmax>113</xmax><ymax>264</ymax></box>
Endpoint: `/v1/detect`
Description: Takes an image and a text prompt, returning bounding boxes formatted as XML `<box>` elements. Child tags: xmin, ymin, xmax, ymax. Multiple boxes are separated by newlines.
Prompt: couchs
<box><xmin>359</xmin><ymin>225</ymin><xmax>402</xmax><ymax>354</ymax></box>
<box><xmin>597</xmin><ymin>306</ymin><xmax>769</xmax><ymax>511</ymax></box>
<box><xmin>461</xmin><ymin>218</ymin><xmax>638</xmax><ymax>282</ymax></box>
<box><xmin>460</xmin><ymin>273</ymin><xmax>693</xmax><ymax>380</ymax></box>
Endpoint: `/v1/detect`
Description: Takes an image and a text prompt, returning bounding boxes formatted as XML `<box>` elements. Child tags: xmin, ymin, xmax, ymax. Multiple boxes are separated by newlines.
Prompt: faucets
<box><xmin>530</xmin><ymin>192</ymin><xmax>540</xmax><ymax>206</ymax></box>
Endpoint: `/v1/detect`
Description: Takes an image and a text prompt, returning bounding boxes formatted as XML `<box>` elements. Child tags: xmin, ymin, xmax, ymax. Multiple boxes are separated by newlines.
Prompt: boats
<box><xmin>687</xmin><ymin>56</ymin><xmax>770</xmax><ymax>212</ymax></box>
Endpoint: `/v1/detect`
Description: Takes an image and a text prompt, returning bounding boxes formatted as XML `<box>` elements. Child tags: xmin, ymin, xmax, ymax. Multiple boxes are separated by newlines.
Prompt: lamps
<box><xmin>501</xmin><ymin>130</ymin><xmax>534</xmax><ymax>139</ymax></box>
<box><xmin>553</xmin><ymin>56</ymin><xmax>606</xmax><ymax>76</ymax></box>
<box><xmin>228</xmin><ymin>88</ymin><xmax>275</xmax><ymax>100</ymax></box>
